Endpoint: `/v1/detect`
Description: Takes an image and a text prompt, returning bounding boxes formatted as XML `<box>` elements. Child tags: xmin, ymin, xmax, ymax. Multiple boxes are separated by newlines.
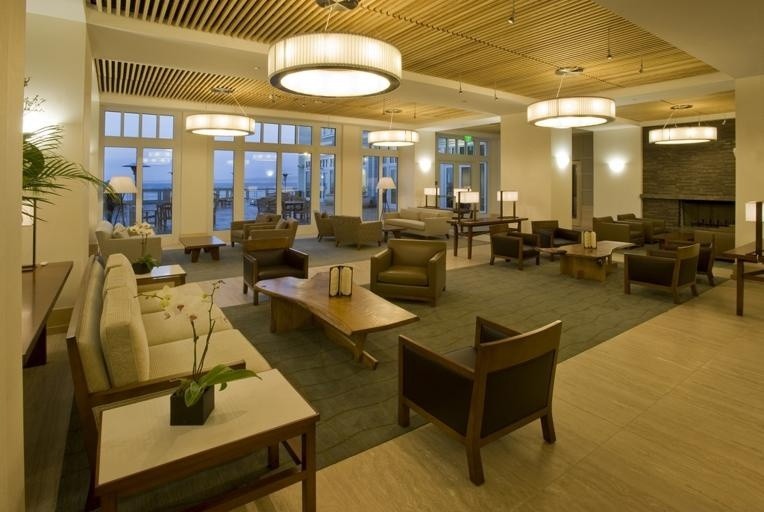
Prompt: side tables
<box><xmin>94</xmin><ymin>367</ymin><xmax>321</xmax><ymax>512</ymax></box>
<box><xmin>135</xmin><ymin>263</ymin><xmax>187</xmax><ymax>292</ymax></box>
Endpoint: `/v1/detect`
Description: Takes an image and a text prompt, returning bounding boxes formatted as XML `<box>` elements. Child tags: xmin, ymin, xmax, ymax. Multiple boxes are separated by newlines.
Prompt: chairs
<box><xmin>624</xmin><ymin>242</ymin><xmax>700</xmax><ymax>304</ymax></box>
<box><xmin>158</xmin><ymin>203</ymin><xmax>171</xmax><ymax>232</ymax></box>
<box><xmin>662</xmin><ymin>234</ymin><xmax>716</xmax><ymax>284</ymax></box>
<box><xmin>531</xmin><ymin>220</ymin><xmax>581</xmax><ymax>262</ymax></box>
<box><xmin>315</xmin><ymin>212</ymin><xmax>352</xmax><ymax>243</ymax></box>
<box><xmin>331</xmin><ymin>215</ymin><xmax>383</xmax><ymax>250</ymax></box>
<box><xmin>593</xmin><ymin>212</ymin><xmax>664</xmax><ymax>245</ymax></box>
<box><xmin>489</xmin><ymin>223</ymin><xmax>540</xmax><ymax>270</ymax></box>
<box><xmin>696</xmin><ymin>228</ymin><xmax>734</xmax><ymax>259</ymax></box>
<box><xmin>241</xmin><ymin>237</ymin><xmax>309</xmax><ymax>305</ymax></box>
<box><xmin>396</xmin><ymin>314</ymin><xmax>563</xmax><ymax>486</ymax></box>
<box><xmin>231</xmin><ymin>214</ymin><xmax>298</xmax><ymax>249</ymax></box>
<box><xmin>370</xmin><ymin>239</ymin><xmax>447</xmax><ymax>308</ymax></box>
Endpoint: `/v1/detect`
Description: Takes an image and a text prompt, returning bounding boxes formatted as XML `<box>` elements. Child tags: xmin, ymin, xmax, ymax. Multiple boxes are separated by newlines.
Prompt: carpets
<box><xmin>160</xmin><ymin>237</ymin><xmax>490</xmax><ymax>282</ymax></box>
<box><xmin>55</xmin><ymin>254</ymin><xmax>730</xmax><ymax>512</ymax></box>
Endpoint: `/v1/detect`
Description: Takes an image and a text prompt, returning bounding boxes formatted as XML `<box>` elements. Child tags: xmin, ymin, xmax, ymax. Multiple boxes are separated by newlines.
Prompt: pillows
<box><xmin>100</xmin><ymin>251</ymin><xmax>149</xmax><ymax>389</ymax></box>
<box><xmin>113</xmin><ymin>222</ymin><xmax>129</xmax><ymax>238</ymax></box>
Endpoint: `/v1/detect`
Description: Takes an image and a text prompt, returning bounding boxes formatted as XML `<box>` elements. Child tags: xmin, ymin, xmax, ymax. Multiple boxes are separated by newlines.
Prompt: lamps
<box><xmin>375</xmin><ymin>176</ymin><xmax>396</xmax><ymax>212</ymax></box>
<box><xmin>744</xmin><ymin>200</ymin><xmax>764</xmax><ymax>256</ymax></box>
<box><xmin>184</xmin><ymin>87</ymin><xmax>256</xmax><ymax>138</ymax></box>
<box><xmin>525</xmin><ymin>66</ymin><xmax>616</xmax><ymax>130</ymax></box>
<box><xmin>420</xmin><ymin>187</ymin><xmax>521</xmax><ymax>221</ymax></box>
<box><xmin>103</xmin><ymin>177</ymin><xmax>139</xmax><ymax>226</ymax></box>
<box><xmin>266</xmin><ymin>0</ymin><xmax>402</xmax><ymax>99</ymax></box>
<box><xmin>647</xmin><ymin>104</ymin><xmax>718</xmax><ymax>146</ymax></box>
<box><xmin>367</xmin><ymin>107</ymin><xmax>419</xmax><ymax>148</ymax></box>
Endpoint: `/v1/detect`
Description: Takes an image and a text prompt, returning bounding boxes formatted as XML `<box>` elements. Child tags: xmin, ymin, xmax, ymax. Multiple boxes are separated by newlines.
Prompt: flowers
<box><xmin>128</xmin><ymin>280</ymin><xmax>263</xmax><ymax>406</ymax></box>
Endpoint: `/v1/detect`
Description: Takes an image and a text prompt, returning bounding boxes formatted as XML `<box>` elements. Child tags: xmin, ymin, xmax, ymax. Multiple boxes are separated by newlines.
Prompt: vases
<box><xmin>169</xmin><ymin>384</ymin><xmax>214</xmax><ymax>425</ymax></box>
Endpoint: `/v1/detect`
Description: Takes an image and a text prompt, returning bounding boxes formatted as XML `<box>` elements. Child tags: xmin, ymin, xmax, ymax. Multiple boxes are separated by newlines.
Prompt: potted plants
<box><xmin>131</xmin><ymin>255</ymin><xmax>159</xmax><ymax>275</ymax></box>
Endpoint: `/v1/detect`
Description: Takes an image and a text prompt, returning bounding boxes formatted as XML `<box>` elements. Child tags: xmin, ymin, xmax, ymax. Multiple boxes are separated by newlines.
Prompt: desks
<box><xmin>724</xmin><ymin>240</ymin><xmax>764</xmax><ymax>316</ymax></box>
<box><xmin>256</xmin><ymin>271</ymin><xmax>420</xmax><ymax>372</ymax></box>
<box><xmin>450</xmin><ymin>217</ymin><xmax>528</xmax><ymax>259</ymax></box>
<box><xmin>382</xmin><ymin>224</ymin><xmax>406</xmax><ymax>242</ymax></box>
<box><xmin>537</xmin><ymin>240</ymin><xmax>634</xmax><ymax>280</ymax></box>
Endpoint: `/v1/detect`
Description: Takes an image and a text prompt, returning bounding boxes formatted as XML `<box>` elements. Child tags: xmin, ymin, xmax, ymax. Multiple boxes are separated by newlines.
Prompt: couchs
<box><xmin>383</xmin><ymin>209</ymin><xmax>453</xmax><ymax>242</ymax></box>
<box><xmin>64</xmin><ymin>255</ymin><xmax>272</xmax><ymax>475</ymax></box>
<box><xmin>95</xmin><ymin>219</ymin><xmax>162</xmax><ymax>269</ymax></box>
